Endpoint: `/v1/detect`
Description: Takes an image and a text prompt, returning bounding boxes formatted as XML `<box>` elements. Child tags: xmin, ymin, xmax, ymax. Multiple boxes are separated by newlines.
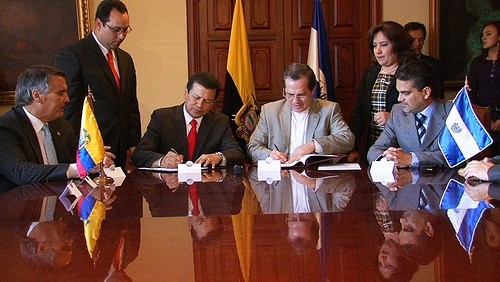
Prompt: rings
<box><xmin>208</xmin><ymin>175</ymin><xmax>212</xmax><ymax>179</ymax></box>
<box><xmin>273</xmin><ymin>156</ymin><xmax>276</xmax><ymax>157</ymax></box>
<box><xmin>388</xmin><ymin>148</ymin><xmax>389</xmax><ymax>152</ymax></box>
<box><xmin>208</xmin><ymin>158</ymin><xmax>212</xmax><ymax>162</ymax></box>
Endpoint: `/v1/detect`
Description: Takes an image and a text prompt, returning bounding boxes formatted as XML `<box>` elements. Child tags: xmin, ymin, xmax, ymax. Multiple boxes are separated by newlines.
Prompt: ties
<box><xmin>415</xmin><ymin>112</ymin><xmax>427</xmax><ymax>145</ymax></box>
<box><xmin>189</xmin><ymin>183</ymin><xmax>200</xmax><ymax>217</ymax></box>
<box><xmin>188</xmin><ymin>120</ymin><xmax>198</xmax><ymax>160</ymax></box>
<box><xmin>419</xmin><ymin>189</ymin><xmax>428</xmax><ymax>209</ymax></box>
<box><xmin>41</xmin><ymin>124</ymin><xmax>58</xmax><ymax>165</ymax></box>
<box><xmin>106</xmin><ymin>50</ymin><xmax>120</xmax><ymax>89</ymax></box>
<box><xmin>45</xmin><ymin>196</ymin><xmax>56</xmax><ymax>222</ymax></box>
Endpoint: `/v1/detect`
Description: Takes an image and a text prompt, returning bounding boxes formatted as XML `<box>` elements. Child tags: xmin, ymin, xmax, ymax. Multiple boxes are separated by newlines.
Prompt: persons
<box><xmin>14</xmin><ymin>173</ymin><xmax>500</xmax><ymax>282</ymax></box>
<box><xmin>403</xmin><ymin>22</ymin><xmax>445</xmax><ymax>99</ymax></box>
<box><xmin>349</xmin><ymin>21</ymin><xmax>436</xmax><ymax>152</ymax></box>
<box><xmin>457</xmin><ymin>155</ymin><xmax>500</xmax><ymax>183</ymax></box>
<box><xmin>0</xmin><ymin>65</ymin><xmax>116</xmax><ymax>193</ymax></box>
<box><xmin>247</xmin><ymin>62</ymin><xmax>356</xmax><ymax>164</ymax></box>
<box><xmin>50</xmin><ymin>0</ymin><xmax>141</xmax><ymax>173</ymax></box>
<box><xmin>132</xmin><ymin>71</ymin><xmax>247</xmax><ymax>172</ymax></box>
<box><xmin>464</xmin><ymin>20</ymin><xmax>500</xmax><ymax>157</ymax></box>
<box><xmin>367</xmin><ymin>61</ymin><xmax>464</xmax><ymax>169</ymax></box>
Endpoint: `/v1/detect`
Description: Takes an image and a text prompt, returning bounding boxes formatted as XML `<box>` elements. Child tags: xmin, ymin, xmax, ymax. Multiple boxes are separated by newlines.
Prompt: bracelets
<box><xmin>216</xmin><ymin>152</ymin><xmax>223</xmax><ymax>159</ymax></box>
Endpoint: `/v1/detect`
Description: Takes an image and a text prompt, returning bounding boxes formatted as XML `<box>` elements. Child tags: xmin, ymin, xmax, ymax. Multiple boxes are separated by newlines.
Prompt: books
<box><xmin>265</xmin><ymin>153</ymin><xmax>338</xmax><ymax>169</ymax></box>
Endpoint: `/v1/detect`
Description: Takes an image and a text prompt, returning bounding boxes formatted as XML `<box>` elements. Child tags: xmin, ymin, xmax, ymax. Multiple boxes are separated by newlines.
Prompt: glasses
<box><xmin>283</xmin><ymin>86</ymin><xmax>315</xmax><ymax>101</ymax></box>
<box><xmin>286</xmin><ymin>216</ymin><xmax>312</xmax><ymax>226</ymax></box>
<box><xmin>102</xmin><ymin>21</ymin><xmax>132</xmax><ymax>34</ymax></box>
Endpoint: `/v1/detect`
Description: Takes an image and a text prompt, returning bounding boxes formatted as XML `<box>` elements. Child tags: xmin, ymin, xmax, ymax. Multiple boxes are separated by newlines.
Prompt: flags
<box><xmin>439</xmin><ymin>179</ymin><xmax>495</xmax><ymax>256</ymax></box>
<box><xmin>75</xmin><ymin>96</ymin><xmax>108</xmax><ymax>180</ymax></box>
<box><xmin>232</xmin><ymin>180</ymin><xmax>257</xmax><ymax>281</ymax></box>
<box><xmin>306</xmin><ymin>0</ymin><xmax>337</xmax><ymax>102</ymax></box>
<box><xmin>222</xmin><ymin>0</ymin><xmax>258</xmax><ymax>148</ymax></box>
<box><xmin>76</xmin><ymin>196</ymin><xmax>107</xmax><ymax>259</ymax></box>
<box><xmin>437</xmin><ymin>88</ymin><xmax>493</xmax><ymax>168</ymax></box>
<box><xmin>314</xmin><ymin>212</ymin><xmax>330</xmax><ymax>282</ymax></box>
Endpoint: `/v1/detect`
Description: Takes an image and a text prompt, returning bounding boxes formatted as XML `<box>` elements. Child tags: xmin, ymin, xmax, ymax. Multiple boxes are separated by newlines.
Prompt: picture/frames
<box><xmin>0</xmin><ymin>0</ymin><xmax>91</xmax><ymax>104</ymax></box>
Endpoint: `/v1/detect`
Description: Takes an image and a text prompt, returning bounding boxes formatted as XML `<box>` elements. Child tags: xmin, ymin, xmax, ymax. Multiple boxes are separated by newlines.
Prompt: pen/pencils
<box><xmin>171</xmin><ymin>148</ymin><xmax>178</xmax><ymax>154</ymax></box>
<box><xmin>274</xmin><ymin>144</ymin><xmax>280</xmax><ymax>151</ymax></box>
<box><xmin>172</xmin><ymin>186</ymin><xmax>178</xmax><ymax>192</ymax></box>
<box><xmin>275</xmin><ymin>181</ymin><xmax>279</xmax><ymax>186</ymax></box>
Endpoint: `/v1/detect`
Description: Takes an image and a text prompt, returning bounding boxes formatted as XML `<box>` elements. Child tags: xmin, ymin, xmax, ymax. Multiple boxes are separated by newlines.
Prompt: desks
<box><xmin>0</xmin><ymin>161</ymin><xmax>500</xmax><ymax>282</ymax></box>
